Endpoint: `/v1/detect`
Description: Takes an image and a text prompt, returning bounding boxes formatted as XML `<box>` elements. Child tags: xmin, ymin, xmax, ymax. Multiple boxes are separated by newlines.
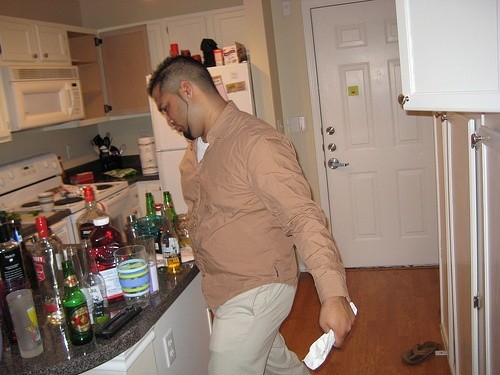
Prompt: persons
<box><xmin>147</xmin><ymin>53</ymin><xmax>356</xmax><ymax>375</ymax></box>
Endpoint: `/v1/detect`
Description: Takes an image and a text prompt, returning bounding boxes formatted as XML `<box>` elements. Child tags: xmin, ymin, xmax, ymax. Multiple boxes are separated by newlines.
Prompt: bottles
<box><xmin>170</xmin><ymin>43</ymin><xmax>201</xmax><ymax>64</ymax></box>
<box><xmin>89</xmin><ymin>217</ymin><xmax>129</xmax><ymax>304</ymax></box>
<box><xmin>124</xmin><ymin>215</ymin><xmax>137</xmax><ymax>246</ymax></box>
<box><xmin>162</xmin><ymin>191</ymin><xmax>178</xmax><ymax>227</ymax></box>
<box><xmin>0</xmin><ymin>215</ymin><xmax>112</xmax><ymax>347</ymax></box>
<box><xmin>145</xmin><ymin>192</ymin><xmax>156</xmax><ymax>215</ymax></box>
<box><xmin>155</xmin><ymin>227</ymin><xmax>163</xmax><ymax>252</ymax></box>
<box><xmin>138</xmin><ymin>136</ymin><xmax>158</xmax><ymax>175</ymax></box>
<box><xmin>75</xmin><ymin>187</ymin><xmax>109</xmax><ymax>242</ymax></box>
<box><xmin>159</xmin><ymin>203</ymin><xmax>182</xmax><ymax>275</ymax></box>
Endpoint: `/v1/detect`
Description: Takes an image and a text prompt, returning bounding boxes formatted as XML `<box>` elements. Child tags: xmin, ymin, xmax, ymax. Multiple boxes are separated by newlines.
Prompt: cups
<box><xmin>6</xmin><ymin>289</ymin><xmax>44</xmax><ymax>359</ymax></box>
<box><xmin>134</xmin><ymin>214</ymin><xmax>161</xmax><ymax>240</ymax></box>
<box><xmin>174</xmin><ymin>214</ymin><xmax>191</xmax><ymax>249</ymax></box>
<box><xmin>99</xmin><ymin>154</ymin><xmax>123</xmax><ymax>171</ymax></box>
<box><xmin>114</xmin><ymin>245</ymin><xmax>152</xmax><ymax>309</ymax></box>
<box><xmin>130</xmin><ymin>234</ymin><xmax>161</xmax><ymax>295</ymax></box>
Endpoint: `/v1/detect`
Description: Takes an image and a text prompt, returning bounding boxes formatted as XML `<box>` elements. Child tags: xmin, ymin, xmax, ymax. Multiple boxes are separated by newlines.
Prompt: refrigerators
<box><xmin>145</xmin><ymin>60</ymin><xmax>258</xmax><ymax>216</ymax></box>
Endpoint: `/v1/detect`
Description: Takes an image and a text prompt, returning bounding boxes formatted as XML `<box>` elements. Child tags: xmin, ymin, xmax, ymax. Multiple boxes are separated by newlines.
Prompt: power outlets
<box><xmin>163</xmin><ymin>328</ymin><xmax>177</xmax><ymax>368</ymax></box>
<box><xmin>66</xmin><ymin>143</ymin><xmax>73</xmax><ymax>159</ymax></box>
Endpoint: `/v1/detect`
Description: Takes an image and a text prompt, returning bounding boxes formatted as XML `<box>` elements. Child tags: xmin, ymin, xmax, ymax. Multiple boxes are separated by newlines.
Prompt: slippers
<box><xmin>401</xmin><ymin>340</ymin><xmax>440</xmax><ymax>365</ymax></box>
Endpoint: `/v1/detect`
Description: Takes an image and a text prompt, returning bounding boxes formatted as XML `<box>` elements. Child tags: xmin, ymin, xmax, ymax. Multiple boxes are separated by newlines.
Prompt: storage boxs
<box><xmin>222</xmin><ymin>41</ymin><xmax>248</xmax><ymax>65</ymax></box>
<box><xmin>213</xmin><ymin>48</ymin><xmax>224</xmax><ymax>66</ymax></box>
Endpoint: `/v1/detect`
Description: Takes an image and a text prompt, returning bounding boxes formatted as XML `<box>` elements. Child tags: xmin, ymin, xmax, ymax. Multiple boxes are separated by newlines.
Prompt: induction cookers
<box><xmin>0</xmin><ymin>152</ymin><xmax>129</xmax><ymax>215</ymax></box>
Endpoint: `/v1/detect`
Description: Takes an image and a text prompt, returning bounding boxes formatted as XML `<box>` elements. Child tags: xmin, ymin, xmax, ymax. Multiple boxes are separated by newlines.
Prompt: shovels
<box><xmin>93</xmin><ymin>134</ymin><xmax>102</xmax><ymax>147</ymax></box>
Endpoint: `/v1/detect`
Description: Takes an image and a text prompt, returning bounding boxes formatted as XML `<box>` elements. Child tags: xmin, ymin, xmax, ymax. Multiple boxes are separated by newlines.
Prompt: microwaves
<box><xmin>0</xmin><ymin>64</ymin><xmax>84</xmax><ymax>133</ymax></box>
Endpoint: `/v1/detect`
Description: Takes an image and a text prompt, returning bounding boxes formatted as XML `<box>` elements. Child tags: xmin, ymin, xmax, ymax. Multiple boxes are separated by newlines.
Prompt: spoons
<box><xmin>91</xmin><ymin>134</ymin><xmax>126</xmax><ymax>154</ymax></box>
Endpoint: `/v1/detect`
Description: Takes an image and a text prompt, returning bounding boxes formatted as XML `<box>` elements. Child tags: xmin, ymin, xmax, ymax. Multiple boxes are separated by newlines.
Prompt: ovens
<box><xmin>69</xmin><ymin>187</ymin><xmax>133</xmax><ymax>244</ymax></box>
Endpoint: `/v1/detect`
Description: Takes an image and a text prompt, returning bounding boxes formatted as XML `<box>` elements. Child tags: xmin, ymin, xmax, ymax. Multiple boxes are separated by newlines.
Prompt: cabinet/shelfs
<box><xmin>33</xmin><ymin>217</ymin><xmax>75</xmax><ymax>245</ymax></box>
<box><xmin>129</xmin><ymin>184</ymin><xmax>142</xmax><ymax>217</ymax></box>
<box><xmin>0</xmin><ymin>5</ymin><xmax>250</xmax><ymax>131</ymax></box>
<box><xmin>138</xmin><ymin>182</ymin><xmax>162</xmax><ymax>216</ymax></box>
<box><xmin>396</xmin><ymin>0</ymin><xmax>500</xmax><ymax>375</ymax></box>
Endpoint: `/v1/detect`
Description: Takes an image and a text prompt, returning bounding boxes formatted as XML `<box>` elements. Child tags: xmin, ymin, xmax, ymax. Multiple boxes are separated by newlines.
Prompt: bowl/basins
<box><xmin>37</xmin><ymin>192</ymin><xmax>53</xmax><ymax>202</ymax></box>
